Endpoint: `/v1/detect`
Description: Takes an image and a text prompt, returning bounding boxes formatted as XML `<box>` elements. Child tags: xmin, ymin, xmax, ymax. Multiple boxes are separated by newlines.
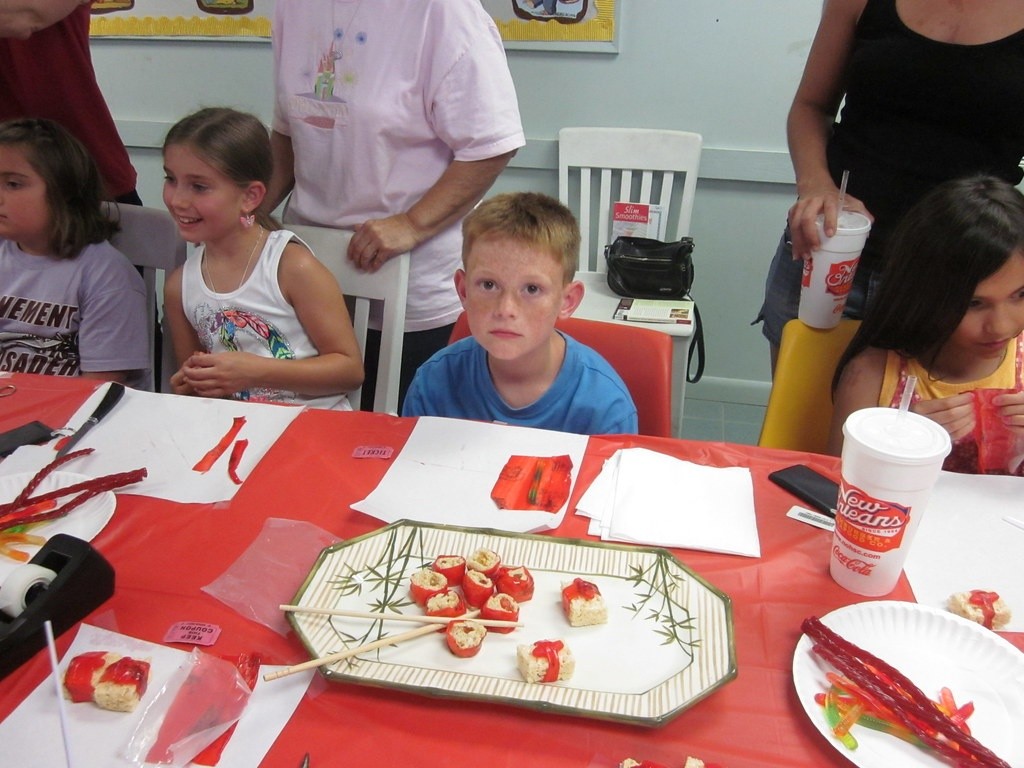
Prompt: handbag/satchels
<box><xmin>604</xmin><ymin>236</ymin><xmax>705</xmax><ymax>383</ymax></box>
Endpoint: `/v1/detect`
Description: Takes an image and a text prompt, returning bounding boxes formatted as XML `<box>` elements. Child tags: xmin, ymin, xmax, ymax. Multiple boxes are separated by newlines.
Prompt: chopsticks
<box><xmin>264</xmin><ymin>604</ymin><xmax>524</xmax><ymax>680</ymax></box>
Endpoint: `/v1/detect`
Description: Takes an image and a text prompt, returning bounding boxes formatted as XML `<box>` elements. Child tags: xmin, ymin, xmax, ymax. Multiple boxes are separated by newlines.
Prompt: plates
<box><xmin>793</xmin><ymin>599</ymin><xmax>1024</xmax><ymax>768</ymax></box>
<box><xmin>285</xmin><ymin>517</ymin><xmax>738</xmax><ymax>729</ymax></box>
<box><xmin>0</xmin><ymin>471</ymin><xmax>117</xmax><ymax>563</ymax></box>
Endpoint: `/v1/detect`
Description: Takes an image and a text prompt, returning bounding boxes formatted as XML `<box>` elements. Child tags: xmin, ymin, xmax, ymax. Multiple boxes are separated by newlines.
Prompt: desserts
<box><xmin>945</xmin><ymin>588</ymin><xmax>1012</xmax><ymax>630</ymax></box>
<box><xmin>409</xmin><ymin>548</ymin><xmax>609</xmax><ymax>684</ymax></box>
<box><xmin>61</xmin><ymin>649</ymin><xmax>151</xmax><ymax>712</ymax></box>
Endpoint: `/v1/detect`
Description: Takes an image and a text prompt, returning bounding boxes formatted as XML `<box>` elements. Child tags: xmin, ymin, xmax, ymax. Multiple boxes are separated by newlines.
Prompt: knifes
<box><xmin>55</xmin><ymin>381</ymin><xmax>126</xmax><ymax>459</ymax></box>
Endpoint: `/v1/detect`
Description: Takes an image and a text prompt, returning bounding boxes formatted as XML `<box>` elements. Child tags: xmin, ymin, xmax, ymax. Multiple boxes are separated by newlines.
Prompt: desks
<box><xmin>0</xmin><ymin>371</ymin><xmax>1024</xmax><ymax>768</ymax></box>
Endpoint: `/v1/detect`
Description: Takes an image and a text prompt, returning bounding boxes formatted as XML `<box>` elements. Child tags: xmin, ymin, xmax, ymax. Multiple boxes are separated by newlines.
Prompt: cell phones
<box><xmin>768</xmin><ymin>464</ymin><xmax>839</xmax><ymax>518</ymax></box>
<box><xmin>0</xmin><ymin>420</ymin><xmax>59</xmax><ymax>459</ymax></box>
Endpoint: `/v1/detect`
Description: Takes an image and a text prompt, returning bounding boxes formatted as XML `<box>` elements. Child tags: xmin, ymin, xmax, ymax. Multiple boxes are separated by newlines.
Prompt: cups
<box><xmin>831</xmin><ymin>407</ymin><xmax>952</xmax><ymax>599</ymax></box>
<box><xmin>798</xmin><ymin>211</ymin><xmax>872</xmax><ymax>330</ymax></box>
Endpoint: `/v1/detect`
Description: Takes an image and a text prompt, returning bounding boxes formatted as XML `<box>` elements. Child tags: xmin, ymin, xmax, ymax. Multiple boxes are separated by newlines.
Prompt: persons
<box><xmin>824</xmin><ymin>176</ymin><xmax>1024</xmax><ymax>477</ymax></box>
<box><xmin>0</xmin><ymin>0</ymin><xmax>162</xmax><ymax>392</ymax></box>
<box><xmin>401</xmin><ymin>194</ymin><xmax>637</xmax><ymax>436</ymax></box>
<box><xmin>258</xmin><ymin>0</ymin><xmax>527</xmax><ymax>418</ymax></box>
<box><xmin>161</xmin><ymin>107</ymin><xmax>364</xmax><ymax>411</ymax></box>
<box><xmin>749</xmin><ymin>0</ymin><xmax>1024</xmax><ymax>381</ymax></box>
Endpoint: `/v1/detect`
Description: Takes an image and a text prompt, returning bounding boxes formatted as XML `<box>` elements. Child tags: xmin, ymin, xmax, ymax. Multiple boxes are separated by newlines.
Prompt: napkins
<box><xmin>347</xmin><ymin>415</ymin><xmax>592</xmax><ymax>535</ymax></box>
<box><xmin>46</xmin><ymin>380</ymin><xmax>311</xmax><ymax>503</ymax></box>
<box><xmin>578</xmin><ymin>447</ymin><xmax>764</xmax><ymax>561</ymax></box>
<box><xmin>900</xmin><ymin>470</ymin><xmax>1024</xmax><ymax>634</ymax></box>
<box><xmin>0</xmin><ymin>620</ymin><xmax>319</xmax><ymax>768</ymax></box>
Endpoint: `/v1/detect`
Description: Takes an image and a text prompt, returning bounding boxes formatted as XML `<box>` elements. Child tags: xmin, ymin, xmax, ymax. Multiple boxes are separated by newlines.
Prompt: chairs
<box><xmin>99</xmin><ymin>127</ymin><xmax>705</xmax><ymax>438</ymax></box>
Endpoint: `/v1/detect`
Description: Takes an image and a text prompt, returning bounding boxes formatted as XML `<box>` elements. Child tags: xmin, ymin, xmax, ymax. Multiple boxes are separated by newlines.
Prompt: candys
<box><xmin>797</xmin><ymin>614</ymin><xmax>1012</xmax><ymax>768</ymax></box>
<box><xmin>0</xmin><ymin>448</ymin><xmax>148</xmax><ymax>561</ymax></box>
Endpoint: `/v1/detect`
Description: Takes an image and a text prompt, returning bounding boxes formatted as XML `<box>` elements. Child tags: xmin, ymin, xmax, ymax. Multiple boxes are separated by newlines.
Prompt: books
<box><xmin>613</xmin><ymin>298</ymin><xmax>694</xmax><ymax>324</ymax></box>
